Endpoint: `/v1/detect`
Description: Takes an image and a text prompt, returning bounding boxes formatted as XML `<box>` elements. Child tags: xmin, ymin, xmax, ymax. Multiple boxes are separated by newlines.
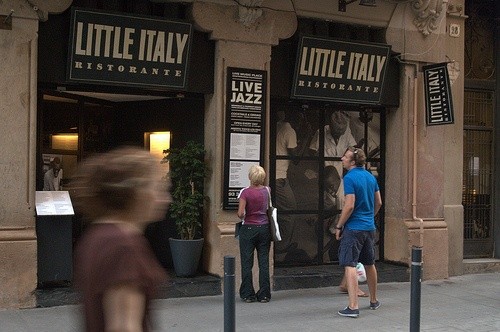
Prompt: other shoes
<box><xmin>341</xmin><ymin>288</ymin><xmax>348</xmax><ymax>292</ymax></box>
<box><xmin>357</xmin><ymin>292</ymin><xmax>368</xmax><ymax>297</ymax></box>
<box><xmin>337</xmin><ymin>306</ymin><xmax>359</xmax><ymax>317</ymax></box>
<box><xmin>261</xmin><ymin>296</ymin><xmax>270</xmax><ymax>302</ymax></box>
<box><xmin>369</xmin><ymin>300</ymin><xmax>381</xmax><ymax>309</ymax></box>
<box><xmin>246</xmin><ymin>296</ymin><xmax>257</xmax><ymax>302</ymax></box>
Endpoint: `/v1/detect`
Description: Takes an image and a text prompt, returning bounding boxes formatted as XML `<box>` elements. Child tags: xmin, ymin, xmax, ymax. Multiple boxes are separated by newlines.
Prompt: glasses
<box><xmin>354</xmin><ymin>148</ymin><xmax>358</xmax><ymax>163</ymax></box>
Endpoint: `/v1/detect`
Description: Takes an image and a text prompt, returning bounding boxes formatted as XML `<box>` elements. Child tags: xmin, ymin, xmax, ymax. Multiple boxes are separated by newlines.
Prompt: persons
<box><xmin>236</xmin><ymin>165</ymin><xmax>273</xmax><ymax>303</ymax></box>
<box><xmin>276</xmin><ymin>105</ymin><xmax>314</xmax><ymax>254</ymax></box>
<box><xmin>43</xmin><ymin>157</ymin><xmax>64</xmax><ymax>190</ymax></box>
<box><xmin>323</xmin><ymin>165</ymin><xmax>345</xmax><ymax>263</ymax></box>
<box><xmin>308</xmin><ymin>112</ymin><xmax>357</xmax><ymax>181</ymax></box>
<box><xmin>334</xmin><ymin>146</ymin><xmax>382</xmax><ymax>317</ymax></box>
<box><xmin>72</xmin><ymin>148</ymin><xmax>171</xmax><ymax>332</ymax></box>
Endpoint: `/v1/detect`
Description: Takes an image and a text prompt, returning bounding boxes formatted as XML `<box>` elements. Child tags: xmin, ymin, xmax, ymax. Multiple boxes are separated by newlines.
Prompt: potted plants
<box><xmin>160</xmin><ymin>139</ymin><xmax>212</xmax><ymax>277</ymax></box>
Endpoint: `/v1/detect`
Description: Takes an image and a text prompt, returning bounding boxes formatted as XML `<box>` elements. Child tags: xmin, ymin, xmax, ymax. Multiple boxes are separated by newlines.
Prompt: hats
<box><xmin>330</xmin><ymin>112</ymin><xmax>347</xmax><ymax>135</ymax></box>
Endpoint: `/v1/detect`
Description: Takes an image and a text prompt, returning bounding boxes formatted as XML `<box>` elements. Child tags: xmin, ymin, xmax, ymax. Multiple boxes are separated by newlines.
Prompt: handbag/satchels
<box><xmin>265</xmin><ymin>187</ymin><xmax>281</xmax><ymax>241</ymax></box>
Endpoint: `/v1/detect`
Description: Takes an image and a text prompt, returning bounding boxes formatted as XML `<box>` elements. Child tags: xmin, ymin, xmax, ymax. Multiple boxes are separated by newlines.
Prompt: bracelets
<box><xmin>335</xmin><ymin>226</ymin><xmax>342</xmax><ymax>231</ymax></box>
